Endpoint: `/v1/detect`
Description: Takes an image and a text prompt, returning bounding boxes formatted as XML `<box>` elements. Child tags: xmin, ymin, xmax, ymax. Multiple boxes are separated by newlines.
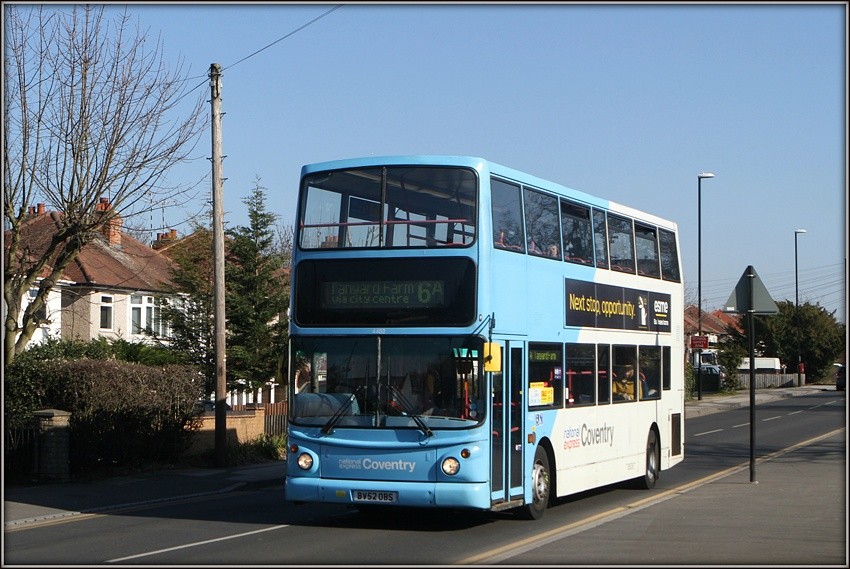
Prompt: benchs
<box><xmin>446</xmin><ymin>241</ymin><xmax>647</xmax><ymax>275</ymax></box>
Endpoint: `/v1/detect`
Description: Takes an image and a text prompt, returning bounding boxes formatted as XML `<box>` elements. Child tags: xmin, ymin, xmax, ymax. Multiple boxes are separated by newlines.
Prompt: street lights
<box><xmin>794</xmin><ymin>229</ymin><xmax>807</xmax><ymax>362</ymax></box>
<box><xmin>698</xmin><ymin>172</ymin><xmax>716</xmax><ymax>399</ymax></box>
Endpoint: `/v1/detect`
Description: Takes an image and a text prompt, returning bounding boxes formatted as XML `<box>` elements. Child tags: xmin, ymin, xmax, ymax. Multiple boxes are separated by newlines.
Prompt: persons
<box><xmin>547</xmin><ymin>244</ymin><xmax>558</xmax><ymax>257</ymax></box>
<box><xmin>612</xmin><ymin>355</ymin><xmax>657</xmax><ymax>401</ymax></box>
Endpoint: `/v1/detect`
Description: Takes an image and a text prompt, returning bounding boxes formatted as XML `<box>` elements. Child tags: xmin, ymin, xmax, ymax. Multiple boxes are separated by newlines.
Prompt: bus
<box><xmin>285</xmin><ymin>153</ymin><xmax>687</xmax><ymax>521</ymax></box>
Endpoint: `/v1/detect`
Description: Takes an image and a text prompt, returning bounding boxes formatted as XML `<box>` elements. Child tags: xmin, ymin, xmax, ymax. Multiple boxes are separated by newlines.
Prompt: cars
<box><xmin>836</xmin><ymin>365</ymin><xmax>846</xmax><ymax>391</ymax></box>
